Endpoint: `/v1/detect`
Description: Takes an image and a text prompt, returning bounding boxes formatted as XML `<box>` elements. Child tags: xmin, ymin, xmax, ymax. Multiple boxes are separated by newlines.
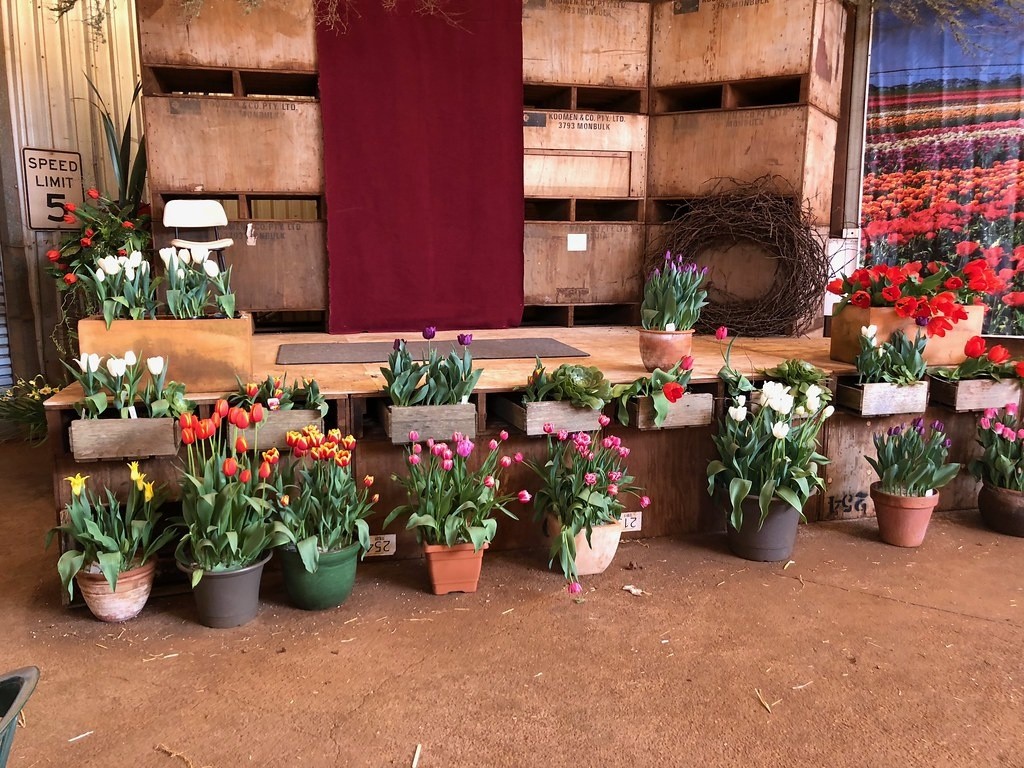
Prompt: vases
<box><xmin>618</xmin><ymin>393</ymin><xmax>712</xmax><ymax>431</ymax></box>
<box><xmin>870</xmin><ymin>481</ymin><xmax>939</xmax><ymax>547</ymax></box>
<box><xmin>838</xmin><ymin>374</ymin><xmax>930</xmax><ymax>418</ymax></box>
<box><xmin>385</xmin><ymin>403</ymin><xmax>476</xmax><ymax>443</ymax></box>
<box><xmin>637</xmin><ymin>327</ymin><xmax>695</xmax><ymax>373</ymax></box>
<box><xmin>978</xmin><ymin>482</ymin><xmax>1024</xmax><ymax>538</ymax></box>
<box><xmin>720</xmin><ymin>483</ymin><xmax>817</xmax><ymax>562</ymax></box>
<box><xmin>928</xmin><ymin>372</ymin><xmax>1020</xmax><ymax>413</ymax></box>
<box><xmin>269</xmin><ymin>538</ymin><xmax>361</xmax><ymax>612</ymax></box>
<box><xmin>78</xmin><ymin>312</ymin><xmax>251</xmax><ymax>391</ymax></box>
<box><xmin>830</xmin><ymin>306</ymin><xmax>984</xmax><ymax>366</ymax></box>
<box><xmin>727</xmin><ymin>380</ymin><xmax>831</xmax><ymax>422</ymax></box>
<box><xmin>176</xmin><ymin>546</ymin><xmax>275</xmax><ymax>628</ymax></box>
<box><xmin>422</xmin><ymin>536</ymin><xmax>489</xmax><ymax>596</ymax></box>
<box><xmin>541</xmin><ymin>508</ymin><xmax>621</xmax><ymax>576</ymax></box>
<box><xmin>227</xmin><ymin>408</ymin><xmax>324</xmax><ymax>450</ymax></box>
<box><xmin>67</xmin><ymin>416</ymin><xmax>181</xmax><ymax>461</ymax></box>
<box><xmin>73</xmin><ymin>549</ymin><xmax>158</xmax><ymax>623</ymax></box>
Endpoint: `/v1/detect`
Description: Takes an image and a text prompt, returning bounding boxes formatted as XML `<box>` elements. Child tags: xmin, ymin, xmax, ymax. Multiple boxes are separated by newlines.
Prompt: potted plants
<box><xmin>72</xmin><ymin>67</ymin><xmax>152</xmax><ymax>231</ymax></box>
<box><xmin>508</xmin><ymin>354</ymin><xmax>613</xmax><ymax>436</ymax></box>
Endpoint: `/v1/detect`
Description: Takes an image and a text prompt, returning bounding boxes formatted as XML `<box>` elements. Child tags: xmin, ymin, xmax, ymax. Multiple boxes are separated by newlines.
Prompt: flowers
<box><xmin>513</xmin><ymin>413</ymin><xmax>650</xmax><ymax>594</ymax></box>
<box><xmin>855</xmin><ymin>324</ymin><xmax>929</xmax><ymax>390</ymax></box>
<box><xmin>706</xmin><ymin>381</ymin><xmax>836</xmax><ymax>534</ymax></box>
<box><xmin>640</xmin><ymin>251</ymin><xmax>710</xmax><ymax>331</ymax></box>
<box><xmin>714</xmin><ymin>325</ymin><xmax>833</xmax><ymax>412</ymax></box>
<box><xmin>44</xmin><ymin>350</ymin><xmax>380</xmax><ymax>601</ymax></box>
<box><xmin>613</xmin><ymin>355</ymin><xmax>695</xmax><ymax>430</ymax></box>
<box><xmin>967</xmin><ymin>403</ymin><xmax>1024</xmax><ymax>491</ymax></box>
<box><xmin>45</xmin><ymin>188</ymin><xmax>151</xmax><ymax>286</ymax></box>
<box><xmin>826</xmin><ymin>261</ymin><xmax>991</xmax><ymax>339</ymax></box>
<box><xmin>863</xmin><ymin>416</ymin><xmax>963</xmax><ymax>498</ymax></box>
<box><xmin>380</xmin><ymin>328</ymin><xmax>484</xmax><ymax>407</ymax></box>
<box><xmin>382</xmin><ymin>429</ymin><xmax>532</xmax><ymax>555</ymax></box>
<box><xmin>77</xmin><ymin>244</ymin><xmax>238</xmax><ymax>331</ymax></box>
<box><xmin>930</xmin><ymin>336</ymin><xmax>1024</xmax><ymax>384</ymax></box>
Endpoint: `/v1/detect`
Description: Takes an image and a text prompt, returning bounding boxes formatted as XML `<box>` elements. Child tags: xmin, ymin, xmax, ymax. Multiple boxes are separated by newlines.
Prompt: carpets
<box><xmin>275</xmin><ymin>337</ymin><xmax>591</xmax><ymax>364</ymax></box>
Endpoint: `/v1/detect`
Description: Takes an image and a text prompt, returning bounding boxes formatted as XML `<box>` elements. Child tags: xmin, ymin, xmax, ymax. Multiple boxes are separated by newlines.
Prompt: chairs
<box><xmin>0</xmin><ymin>665</ymin><xmax>40</xmax><ymax>768</ymax></box>
<box><xmin>163</xmin><ymin>200</ymin><xmax>235</xmax><ymax>271</ymax></box>
